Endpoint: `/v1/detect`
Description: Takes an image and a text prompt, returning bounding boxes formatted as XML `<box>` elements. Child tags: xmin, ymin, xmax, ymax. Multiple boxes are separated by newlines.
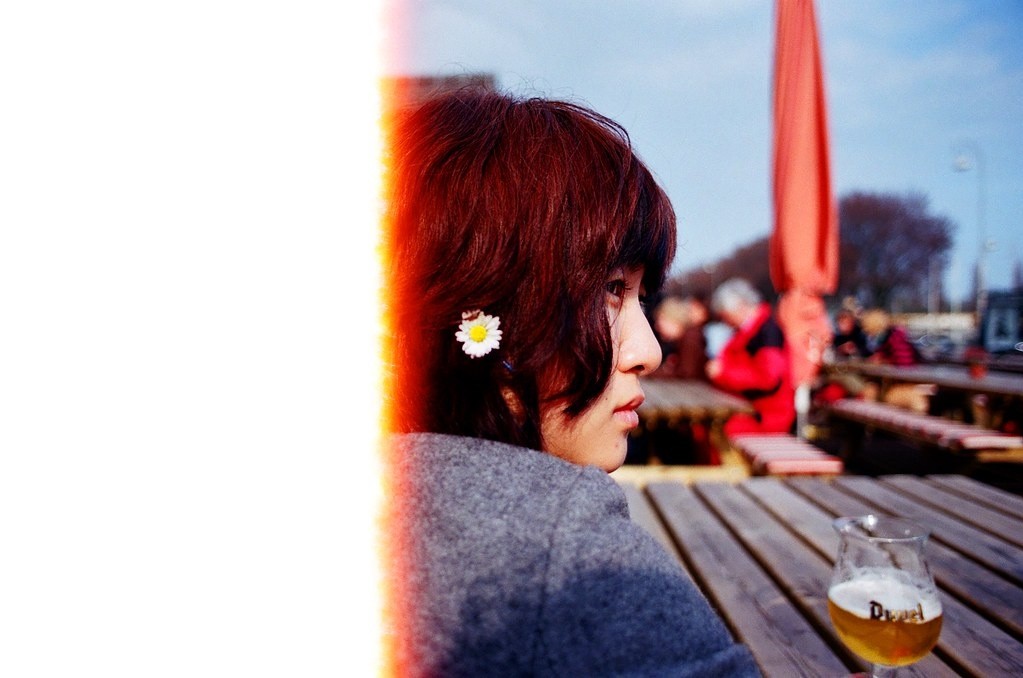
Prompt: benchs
<box><xmin>832</xmin><ymin>398</ymin><xmax>1023</xmax><ymax>465</ymax></box>
<box><xmin>730</xmin><ymin>433</ymin><xmax>843</xmax><ymax>478</ymax></box>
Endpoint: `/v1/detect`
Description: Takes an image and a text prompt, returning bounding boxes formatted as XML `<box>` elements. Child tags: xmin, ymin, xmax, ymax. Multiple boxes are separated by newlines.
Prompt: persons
<box><xmin>658</xmin><ymin>281</ymin><xmax>923</xmax><ymax>442</ymax></box>
<box><xmin>382</xmin><ymin>67</ymin><xmax>766</xmax><ymax>678</ymax></box>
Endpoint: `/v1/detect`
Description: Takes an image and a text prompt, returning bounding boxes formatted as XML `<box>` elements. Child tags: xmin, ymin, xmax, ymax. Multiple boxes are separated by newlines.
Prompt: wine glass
<box><xmin>825</xmin><ymin>515</ymin><xmax>943</xmax><ymax>678</ymax></box>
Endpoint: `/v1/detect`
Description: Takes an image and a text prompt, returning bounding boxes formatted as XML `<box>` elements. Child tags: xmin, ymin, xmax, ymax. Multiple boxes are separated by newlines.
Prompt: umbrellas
<box><xmin>769</xmin><ymin>0</ymin><xmax>839</xmax><ymax>438</ymax></box>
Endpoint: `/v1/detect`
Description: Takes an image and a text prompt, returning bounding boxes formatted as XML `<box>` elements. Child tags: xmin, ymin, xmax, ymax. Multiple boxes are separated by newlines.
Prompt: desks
<box><xmin>629</xmin><ymin>365</ymin><xmax>1023</xmax><ymax>678</ymax></box>
